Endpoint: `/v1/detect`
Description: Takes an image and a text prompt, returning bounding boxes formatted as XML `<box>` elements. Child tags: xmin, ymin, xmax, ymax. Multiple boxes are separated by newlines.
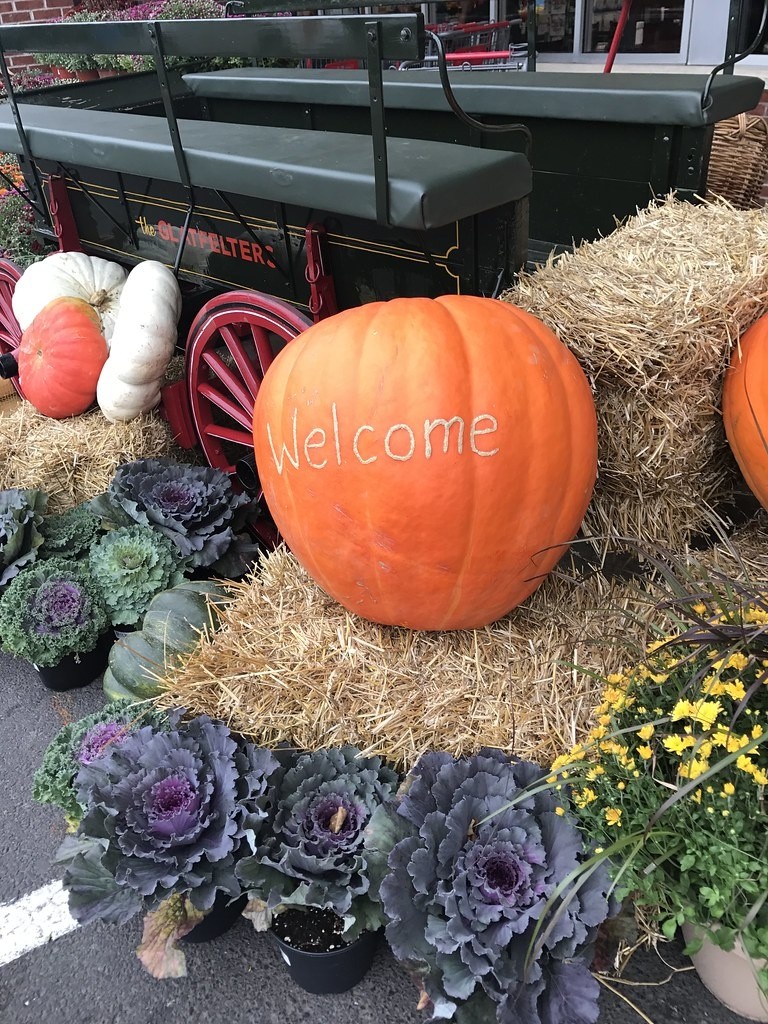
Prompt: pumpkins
<box><xmin>253</xmin><ymin>295</ymin><xmax>598</xmax><ymax>630</ymax></box>
<box><xmin>103</xmin><ymin>579</ymin><xmax>230</xmax><ymax>706</ymax></box>
<box><xmin>722</xmin><ymin>310</ymin><xmax>768</xmax><ymax>516</ymax></box>
<box><xmin>11</xmin><ymin>251</ymin><xmax>181</xmax><ymax>423</ymax></box>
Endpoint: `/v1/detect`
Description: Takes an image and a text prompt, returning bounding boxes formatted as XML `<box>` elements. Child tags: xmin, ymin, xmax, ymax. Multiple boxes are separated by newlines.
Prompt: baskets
<box><xmin>704</xmin><ymin>115</ymin><xmax>768</xmax><ymax>208</ymax></box>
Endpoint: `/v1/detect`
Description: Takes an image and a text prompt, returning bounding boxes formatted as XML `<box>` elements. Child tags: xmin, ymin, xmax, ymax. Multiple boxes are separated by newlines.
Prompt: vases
<box><xmin>50</xmin><ymin>64</ymin><xmax>75</xmax><ymax>81</ymax></box>
<box><xmin>678</xmin><ymin>905</ymin><xmax>768</xmax><ymax>1024</ymax></box>
<box><xmin>115</xmin><ymin>630</ymin><xmax>140</xmax><ymax>642</ymax></box>
<box><xmin>261</xmin><ymin>907</ymin><xmax>373</xmax><ymax>997</ymax></box>
<box><xmin>176</xmin><ymin>890</ymin><xmax>241</xmax><ymax>944</ymax></box>
<box><xmin>32</xmin><ymin>654</ymin><xmax>82</xmax><ymax>692</ymax></box>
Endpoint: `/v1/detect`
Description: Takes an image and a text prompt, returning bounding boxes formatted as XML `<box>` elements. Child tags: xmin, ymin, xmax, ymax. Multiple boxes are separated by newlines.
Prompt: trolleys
<box><xmin>395</xmin><ymin>19</ymin><xmax>539</xmax><ymax>72</ymax></box>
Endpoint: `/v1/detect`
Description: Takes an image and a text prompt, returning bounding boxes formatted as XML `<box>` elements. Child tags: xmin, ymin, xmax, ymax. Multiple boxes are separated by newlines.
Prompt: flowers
<box><xmin>235</xmin><ymin>744</ymin><xmax>392</xmax><ymax>943</ymax></box>
<box><xmin>88</xmin><ymin>524</ymin><xmax>192</xmax><ymax>627</ymax></box>
<box><xmin>371</xmin><ymin>750</ymin><xmax>618</xmax><ymax>1024</ymax></box>
<box><xmin>112</xmin><ymin>460</ymin><xmax>259</xmax><ymax>579</ymax></box>
<box><xmin>56</xmin><ymin>710</ymin><xmax>264</xmax><ymax>976</ymax></box>
<box><xmin>544</xmin><ymin>604</ymin><xmax>768</xmax><ymax>956</ymax></box>
<box><xmin>123</xmin><ymin>0</ymin><xmax>167</xmax><ymax>22</ymax></box>
<box><xmin>0</xmin><ymin>485</ymin><xmax>51</xmax><ymax>586</ymax></box>
<box><xmin>0</xmin><ymin>559</ymin><xmax>110</xmax><ymax>672</ymax></box>
<box><xmin>43</xmin><ymin>16</ymin><xmax>65</xmax><ymax>24</ymax></box>
<box><xmin>41</xmin><ymin>501</ymin><xmax>102</xmax><ymax>559</ymax></box>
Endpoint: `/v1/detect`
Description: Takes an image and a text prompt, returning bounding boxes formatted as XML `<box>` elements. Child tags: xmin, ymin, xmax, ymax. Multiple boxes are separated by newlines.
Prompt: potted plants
<box><xmin>95</xmin><ymin>10</ymin><xmax>127</xmax><ymax>79</ymax></box>
<box><xmin>65</xmin><ymin>11</ymin><xmax>98</xmax><ymax>82</ymax></box>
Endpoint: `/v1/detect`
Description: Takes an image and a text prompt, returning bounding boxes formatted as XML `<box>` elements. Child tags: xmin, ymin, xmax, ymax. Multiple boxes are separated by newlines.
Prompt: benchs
<box><xmin>181</xmin><ymin>0</ymin><xmax>768</xmax><ymax>276</ymax></box>
<box><xmin>0</xmin><ymin>10</ymin><xmax>531</xmax><ymax>300</ymax></box>
